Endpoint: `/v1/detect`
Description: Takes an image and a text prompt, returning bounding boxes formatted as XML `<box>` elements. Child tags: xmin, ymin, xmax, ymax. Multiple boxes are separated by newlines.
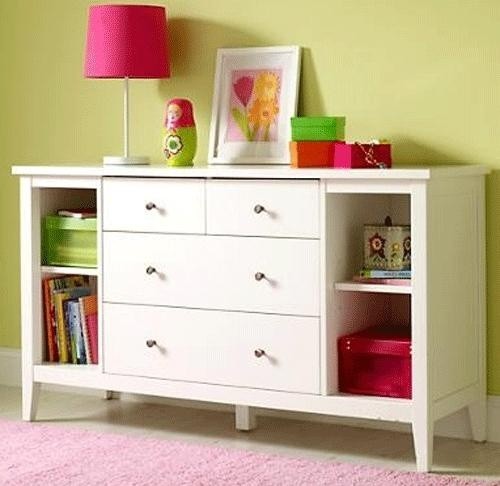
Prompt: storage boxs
<box><xmin>39</xmin><ymin>212</ymin><xmax>96</xmax><ymax>267</ymax></box>
<box><xmin>337</xmin><ymin>326</ymin><xmax>411</xmax><ymax>399</ymax></box>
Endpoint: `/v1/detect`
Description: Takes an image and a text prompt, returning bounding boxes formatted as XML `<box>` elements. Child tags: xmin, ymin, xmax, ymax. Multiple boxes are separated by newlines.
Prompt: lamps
<box><xmin>83</xmin><ymin>5</ymin><xmax>171</xmax><ymax>165</ymax></box>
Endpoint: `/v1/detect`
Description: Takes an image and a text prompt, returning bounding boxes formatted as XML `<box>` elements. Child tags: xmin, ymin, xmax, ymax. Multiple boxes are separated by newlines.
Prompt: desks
<box><xmin>9</xmin><ymin>165</ymin><xmax>490</xmax><ymax>474</ymax></box>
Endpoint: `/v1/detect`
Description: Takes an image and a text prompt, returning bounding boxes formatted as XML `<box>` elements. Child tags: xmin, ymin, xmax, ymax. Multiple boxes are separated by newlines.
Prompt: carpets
<box><xmin>0</xmin><ymin>418</ymin><xmax>500</xmax><ymax>484</ymax></box>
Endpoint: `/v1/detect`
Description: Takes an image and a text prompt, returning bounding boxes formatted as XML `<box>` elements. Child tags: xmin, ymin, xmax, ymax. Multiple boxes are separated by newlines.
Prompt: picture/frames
<box><xmin>208</xmin><ymin>45</ymin><xmax>303</xmax><ymax>166</ymax></box>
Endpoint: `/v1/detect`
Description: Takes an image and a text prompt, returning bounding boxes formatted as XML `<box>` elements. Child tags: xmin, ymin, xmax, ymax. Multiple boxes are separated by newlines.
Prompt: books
<box><xmin>57</xmin><ymin>209</ymin><xmax>97</xmax><ymax>219</ymax></box>
<box><xmin>353</xmin><ymin>270</ymin><xmax>411</xmax><ymax>288</ymax></box>
<box><xmin>42</xmin><ymin>275</ymin><xmax>98</xmax><ymax>364</ymax></box>
